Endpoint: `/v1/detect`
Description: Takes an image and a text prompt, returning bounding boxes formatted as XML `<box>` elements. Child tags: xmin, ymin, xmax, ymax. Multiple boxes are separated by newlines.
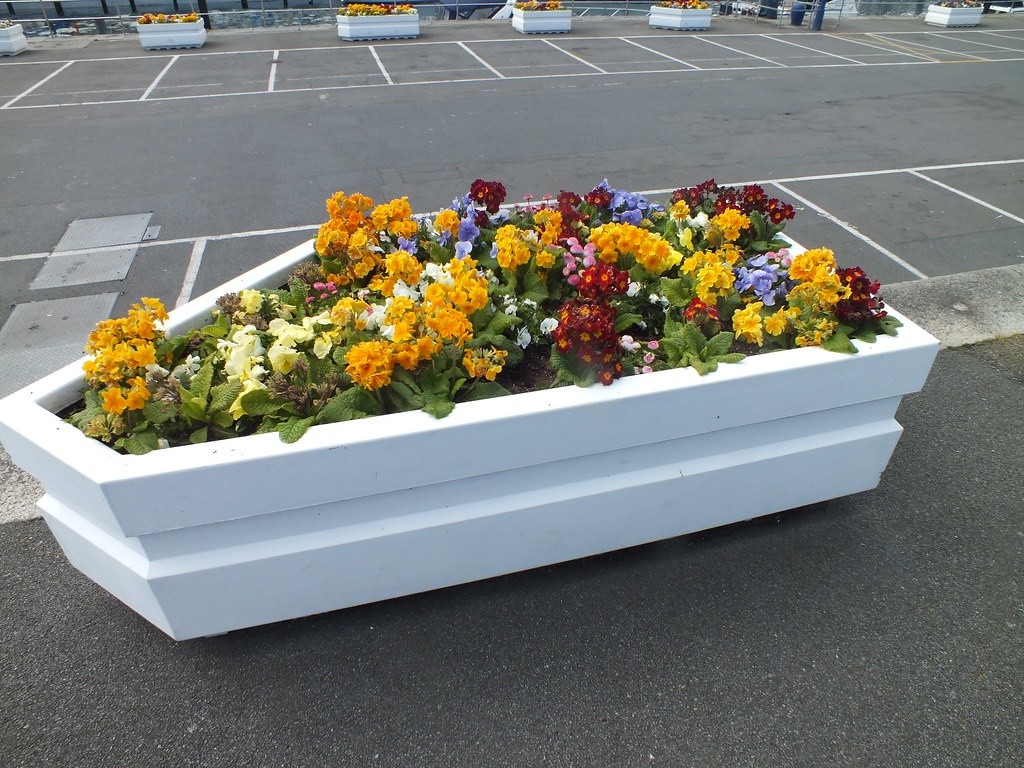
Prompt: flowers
<box><xmin>514</xmin><ymin>0</ymin><xmax>561</xmax><ymax>11</ymax></box>
<box><xmin>0</xmin><ymin>19</ymin><xmax>14</xmax><ymax>29</ymax></box>
<box><xmin>59</xmin><ymin>179</ymin><xmax>904</xmax><ymax>454</ymax></box>
<box><xmin>655</xmin><ymin>0</ymin><xmax>708</xmax><ymax>9</ymax></box>
<box><xmin>338</xmin><ymin>3</ymin><xmax>417</xmax><ymax>16</ymax></box>
<box><xmin>939</xmin><ymin>0</ymin><xmax>980</xmax><ymax>8</ymax></box>
<box><xmin>137</xmin><ymin>12</ymin><xmax>200</xmax><ymax>24</ymax></box>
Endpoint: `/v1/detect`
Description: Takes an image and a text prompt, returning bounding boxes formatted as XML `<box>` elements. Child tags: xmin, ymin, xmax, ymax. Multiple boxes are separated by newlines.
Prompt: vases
<box><xmin>137</xmin><ymin>18</ymin><xmax>206</xmax><ymax>50</ymax></box>
<box><xmin>925</xmin><ymin>4</ymin><xmax>985</xmax><ymax>28</ymax></box>
<box><xmin>1</xmin><ymin>235</ymin><xmax>941</xmax><ymax>641</ymax></box>
<box><xmin>0</xmin><ymin>24</ymin><xmax>28</xmax><ymax>56</ymax></box>
<box><xmin>649</xmin><ymin>6</ymin><xmax>712</xmax><ymax>30</ymax></box>
<box><xmin>512</xmin><ymin>8</ymin><xmax>572</xmax><ymax>35</ymax></box>
<box><xmin>337</xmin><ymin>15</ymin><xmax>420</xmax><ymax>41</ymax></box>
